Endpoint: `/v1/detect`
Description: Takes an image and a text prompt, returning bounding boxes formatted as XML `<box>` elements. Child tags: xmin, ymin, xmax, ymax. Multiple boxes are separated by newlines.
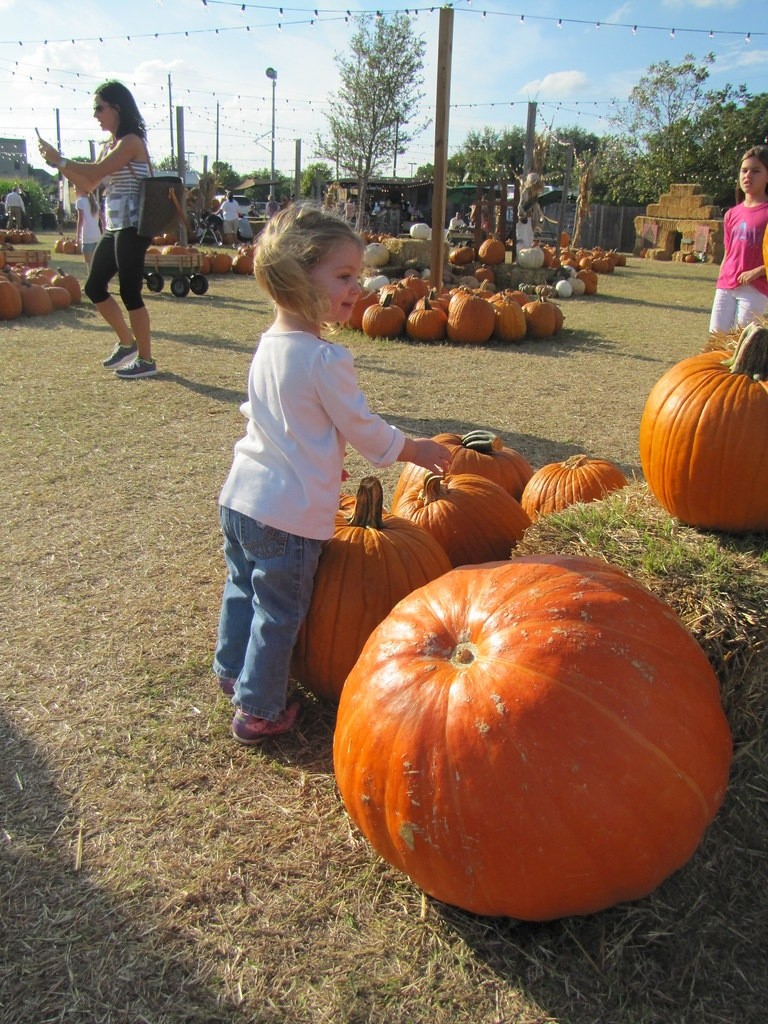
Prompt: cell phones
<box><xmin>35</xmin><ymin>128</ymin><xmax>40</xmax><ymax>139</ymax></box>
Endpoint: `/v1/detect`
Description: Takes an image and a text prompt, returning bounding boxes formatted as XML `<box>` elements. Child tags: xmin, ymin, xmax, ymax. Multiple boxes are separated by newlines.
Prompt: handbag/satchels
<box><xmin>121</xmin><ymin>133</ymin><xmax>187</xmax><ymax>238</ymax></box>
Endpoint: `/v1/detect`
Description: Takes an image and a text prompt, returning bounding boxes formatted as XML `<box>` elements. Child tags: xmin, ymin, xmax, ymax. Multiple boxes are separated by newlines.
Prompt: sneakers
<box><xmin>113</xmin><ymin>357</ymin><xmax>158</xmax><ymax>378</ymax></box>
<box><xmin>102</xmin><ymin>337</ymin><xmax>138</xmax><ymax>368</ymax></box>
<box><xmin>218</xmin><ymin>678</ymin><xmax>236</xmax><ymax>696</ymax></box>
<box><xmin>231</xmin><ymin>695</ymin><xmax>306</xmax><ymax>745</ymax></box>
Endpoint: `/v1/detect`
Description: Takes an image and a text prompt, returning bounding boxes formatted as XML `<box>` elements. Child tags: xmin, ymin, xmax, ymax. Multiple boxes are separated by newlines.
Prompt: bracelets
<box><xmin>59</xmin><ymin>158</ymin><xmax>68</xmax><ymax>168</ymax></box>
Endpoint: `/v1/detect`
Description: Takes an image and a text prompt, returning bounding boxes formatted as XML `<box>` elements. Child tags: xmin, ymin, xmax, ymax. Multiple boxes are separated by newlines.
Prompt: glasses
<box><xmin>93</xmin><ymin>104</ymin><xmax>118</xmax><ymax>112</ymax></box>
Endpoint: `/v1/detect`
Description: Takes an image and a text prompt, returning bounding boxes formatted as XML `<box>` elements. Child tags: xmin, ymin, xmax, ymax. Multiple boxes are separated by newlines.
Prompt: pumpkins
<box><xmin>640</xmin><ymin>250</ymin><xmax>646</xmax><ymax>257</ymax></box>
<box><xmin>685</xmin><ymin>255</ymin><xmax>695</xmax><ymax>262</ymax></box>
<box><xmin>53</xmin><ymin>231</ymin><xmax>259</xmax><ymax>275</ymax></box>
<box><xmin>0</xmin><ymin>227</ymin><xmax>38</xmax><ymax>244</ymax></box>
<box><xmin>287</xmin><ymin>428</ymin><xmax>628</xmax><ymax>704</ymax></box>
<box><xmin>638</xmin><ymin>319</ymin><xmax>768</xmax><ymax>534</ymax></box>
<box><xmin>332</xmin><ymin>551</ymin><xmax>732</xmax><ymax>922</ymax></box>
<box><xmin>346</xmin><ymin>223</ymin><xmax>626</xmax><ymax>343</ymax></box>
<box><xmin>0</xmin><ymin>261</ymin><xmax>82</xmax><ymax>319</ymax></box>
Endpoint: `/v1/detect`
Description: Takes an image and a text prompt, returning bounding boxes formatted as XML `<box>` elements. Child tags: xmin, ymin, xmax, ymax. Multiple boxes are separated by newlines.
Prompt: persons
<box><xmin>710</xmin><ymin>145</ymin><xmax>768</xmax><ymax>333</ymax></box>
<box><xmin>450</xmin><ymin>212</ymin><xmax>464</xmax><ymax>227</ymax></box>
<box><xmin>73</xmin><ymin>184</ymin><xmax>106</xmax><ymax>271</ymax></box>
<box><xmin>344</xmin><ymin>198</ymin><xmax>356</xmax><ymax>222</ymax></box>
<box><xmin>237</xmin><ymin>215</ymin><xmax>252</xmax><ymax>245</ymax></box>
<box><xmin>0</xmin><ymin>184</ymin><xmax>31</xmax><ymax>231</ymax></box>
<box><xmin>215</xmin><ymin>191</ymin><xmax>240</xmax><ymax>249</ymax></box>
<box><xmin>54</xmin><ymin>201</ymin><xmax>66</xmax><ymax>235</ymax></box>
<box><xmin>265</xmin><ymin>195</ymin><xmax>289</xmax><ymax>217</ymax></box>
<box><xmin>214</xmin><ymin>207</ymin><xmax>453</xmax><ymax>743</ymax></box>
<box><xmin>37</xmin><ymin>82</ymin><xmax>157</xmax><ymax>378</ymax></box>
